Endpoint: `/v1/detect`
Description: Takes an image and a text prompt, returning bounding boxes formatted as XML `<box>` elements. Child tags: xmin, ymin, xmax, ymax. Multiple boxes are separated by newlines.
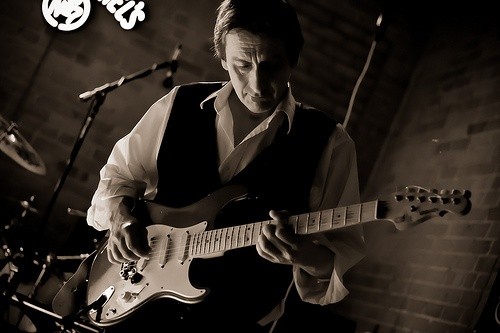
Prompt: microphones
<box><xmin>163</xmin><ymin>45</ymin><xmax>183</xmax><ymax>88</ymax></box>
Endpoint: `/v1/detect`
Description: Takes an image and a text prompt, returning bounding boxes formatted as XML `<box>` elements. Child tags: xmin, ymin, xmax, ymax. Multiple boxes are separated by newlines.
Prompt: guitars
<box><xmin>86</xmin><ymin>184</ymin><xmax>474</xmax><ymax>327</ymax></box>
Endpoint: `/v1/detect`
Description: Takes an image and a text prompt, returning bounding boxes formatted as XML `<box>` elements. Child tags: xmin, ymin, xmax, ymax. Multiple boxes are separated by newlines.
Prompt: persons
<box><xmin>87</xmin><ymin>0</ymin><xmax>366</xmax><ymax>333</ymax></box>
